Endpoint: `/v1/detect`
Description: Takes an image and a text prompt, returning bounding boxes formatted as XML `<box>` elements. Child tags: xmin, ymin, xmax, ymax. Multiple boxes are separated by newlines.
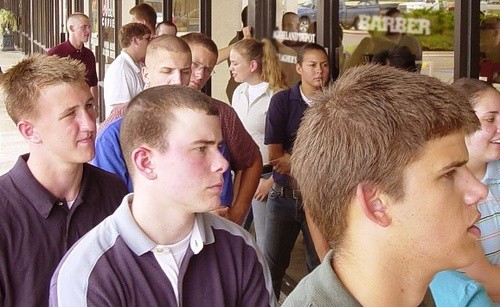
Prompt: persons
<box><xmin>0</xmin><ymin>55</ymin><xmax>135</xmax><ymax>307</ymax></box>
<box><xmin>49</xmin><ymin>12</ymin><xmax>100</xmax><ymax>121</ymax></box>
<box><xmin>152</xmin><ymin>22</ymin><xmax>178</xmax><ymax>41</ymax></box>
<box><xmin>96</xmin><ymin>32</ymin><xmax>263</xmax><ymax>226</ymax></box>
<box><xmin>47</xmin><ymin>84</ymin><xmax>281</xmax><ymax>307</ymax></box>
<box><xmin>104</xmin><ymin>22</ymin><xmax>150</xmax><ymax>121</ymax></box>
<box><xmin>214</xmin><ymin>5</ymin><xmax>252</xmax><ymax>104</ymax></box>
<box><xmin>227</xmin><ymin>38</ymin><xmax>288</xmax><ymax>254</ymax></box>
<box><xmin>280</xmin><ymin>64</ymin><xmax>489</xmax><ymax>307</ymax></box>
<box><xmin>273</xmin><ymin>6</ymin><xmax>499</xmax><ymax>107</ymax></box>
<box><xmin>129</xmin><ymin>3</ymin><xmax>158</xmax><ymax>42</ymax></box>
<box><xmin>91</xmin><ymin>33</ymin><xmax>233</xmax><ymax>221</ymax></box>
<box><xmin>428</xmin><ymin>76</ymin><xmax>499</xmax><ymax>307</ymax></box>
<box><xmin>260</xmin><ymin>43</ymin><xmax>322</xmax><ymax>304</ymax></box>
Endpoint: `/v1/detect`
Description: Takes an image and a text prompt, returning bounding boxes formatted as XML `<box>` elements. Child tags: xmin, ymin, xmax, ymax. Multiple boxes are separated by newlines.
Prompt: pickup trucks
<box><xmin>298</xmin><ymin>0</ymin><xmax>487</xmax><ymax>33</ymax></box>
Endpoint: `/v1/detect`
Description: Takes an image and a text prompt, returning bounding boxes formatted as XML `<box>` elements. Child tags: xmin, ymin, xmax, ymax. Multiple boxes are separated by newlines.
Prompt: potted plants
<box><xmin>0</xmin><ymin>9</ymin><xmax>18</xmax><ymax>51</ymax></box>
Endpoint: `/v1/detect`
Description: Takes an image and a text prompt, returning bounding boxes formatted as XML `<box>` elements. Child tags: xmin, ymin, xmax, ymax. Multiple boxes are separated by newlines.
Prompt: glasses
<box><xmin>142</xmin><ymin>37</ymin><xmax>153</xmax><ymax>41</ymax></box>
<box><xmin>192</xmin><ymin>61</ymin><xmax>215</xmax><ymax>77</ymax></box>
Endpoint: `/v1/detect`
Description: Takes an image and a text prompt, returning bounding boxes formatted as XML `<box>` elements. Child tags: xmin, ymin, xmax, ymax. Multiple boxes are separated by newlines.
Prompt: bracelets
<box><xmin>93</xmin><ymin>105</ymin><xmax>98</xmax><ymax>110</ymax></box>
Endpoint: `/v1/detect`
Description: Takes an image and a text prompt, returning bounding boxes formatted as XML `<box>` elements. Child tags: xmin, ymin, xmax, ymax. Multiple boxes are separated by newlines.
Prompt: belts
<box><xmin>272</xmin><ymin>182</ymin><xmax>303</xmax><ymax>200</ymax></box>
